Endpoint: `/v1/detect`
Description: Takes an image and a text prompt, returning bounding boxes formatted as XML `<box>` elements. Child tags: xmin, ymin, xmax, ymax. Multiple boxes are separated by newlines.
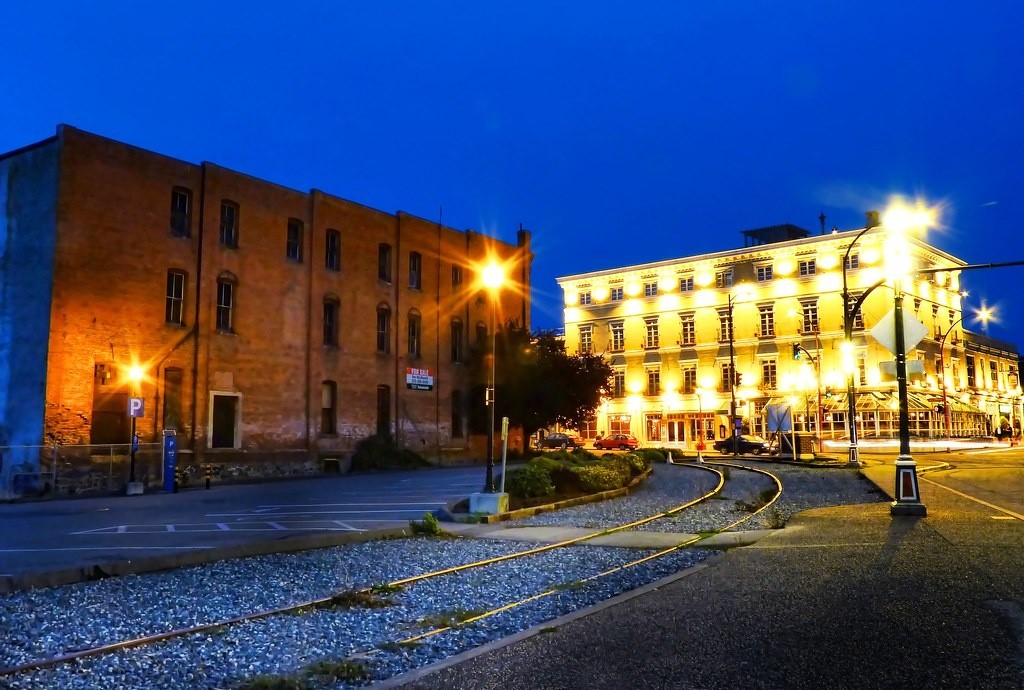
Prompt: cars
<box><xmin>712</xmin><ymin>434</ymin><xmax>771</xmax><ymax>455</ymax></box>
<box><xmin>534</xmin><ymin>431</ymin><xmax>586</xmax><ymax>450</ymax></box>
<box><xmin>593</xmin><ymin>433</ymin><xmax>639</xmax><ymax>451</ymax></box>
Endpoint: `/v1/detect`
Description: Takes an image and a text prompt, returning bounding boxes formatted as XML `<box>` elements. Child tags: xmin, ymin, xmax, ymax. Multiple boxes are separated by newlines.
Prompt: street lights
<box><xmin>469</xmin><ymin>260</ymin><xmax>510</xmax><ymax>515</ymax></box>
<box><xmin>940</xmin><ymin>308</ymin><xmax>989</xmax><ymax>441</ymax></box>
<box><xmin>125</xmin><ymin>365</ymin><xmax>145</xmax><ymax>495</ymax></box>
<box><xmin>840</xmin><ymin>205</ymin><xmax>911</xmax><ymax>467</ymax></box>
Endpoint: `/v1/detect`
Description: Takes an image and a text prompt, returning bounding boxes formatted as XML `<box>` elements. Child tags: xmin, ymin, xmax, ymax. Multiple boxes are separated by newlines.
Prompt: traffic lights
<box><xmin>792</xmin><ymin>342</ymin><xmax>801</xmax><ymax>360</ymax></box>
